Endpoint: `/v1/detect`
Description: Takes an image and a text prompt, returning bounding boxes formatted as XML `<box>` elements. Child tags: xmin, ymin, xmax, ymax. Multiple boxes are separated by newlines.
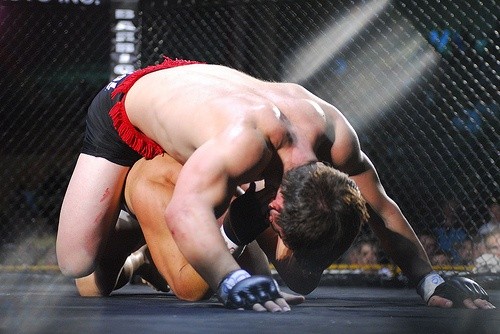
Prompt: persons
<box><xmin>55</xmin><ymin>54</ymin><xmax>500</xmax><ymax>311</ymax></box>
